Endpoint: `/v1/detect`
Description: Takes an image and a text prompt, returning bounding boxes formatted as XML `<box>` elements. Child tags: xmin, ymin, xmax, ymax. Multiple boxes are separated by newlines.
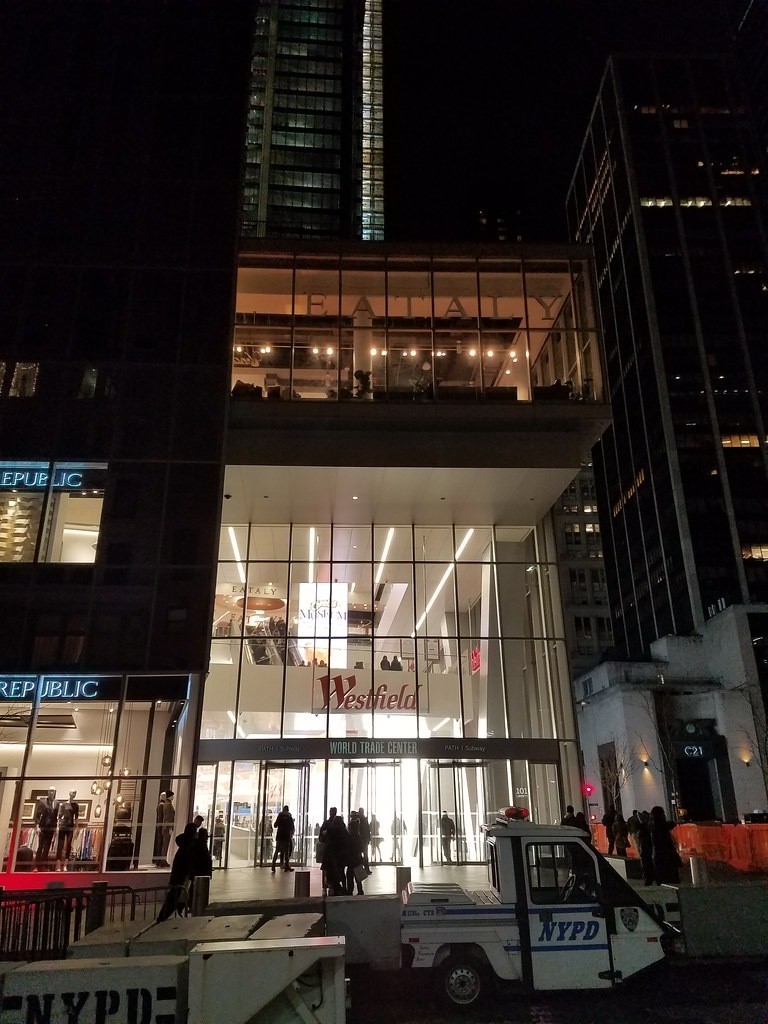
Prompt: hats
<box><xmin>165</xmin><ymin>790</ymin><xmax>174</xmax><ymax>798</ymax></box>
<box><xmin>283</xmin><ymin>805</ymin><xmax>290</xmax><ymax>812</ymax></box>
<box><xmin>350</xmin><ymin>810</ymin><xmax>357</xmax><ymax>816</ymax></box>
<box><xmin>637</xmin><ymin>810</ymin><xmax>650</xmax><ymax>825</ymax></box>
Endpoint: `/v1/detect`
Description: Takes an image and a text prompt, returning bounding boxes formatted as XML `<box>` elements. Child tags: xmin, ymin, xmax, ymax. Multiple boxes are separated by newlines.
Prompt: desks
<box><xmin>222</xmin><ymin>822</ymin><xmax>258</xmax><ymax>862</ymax></box>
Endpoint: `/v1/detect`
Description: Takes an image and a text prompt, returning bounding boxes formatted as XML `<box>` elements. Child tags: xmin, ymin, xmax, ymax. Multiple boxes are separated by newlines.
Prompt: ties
<box><xmin>50</xmin><ymin>801</ymin><xmax>53</xmax><ymax>808</ymax></box>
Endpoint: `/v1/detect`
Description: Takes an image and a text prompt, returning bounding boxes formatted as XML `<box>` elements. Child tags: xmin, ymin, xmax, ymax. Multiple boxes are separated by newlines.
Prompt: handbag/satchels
<box><xmin>624</xmin><ymin>838</ymin><xmax>631</xmax><ymax>848</ymax></box>
<box><xmin>353</xmin><ymin>864</ymin><xmax>369</xmax><ymax>882</ymax></box>
<box><xmin>315</xmin><ymin>843</ymin><xmax>326</xmax><ymax>863</ymax></box>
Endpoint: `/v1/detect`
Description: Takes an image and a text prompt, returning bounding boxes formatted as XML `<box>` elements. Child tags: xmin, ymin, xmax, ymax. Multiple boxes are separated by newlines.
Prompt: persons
<box><xmin>313</xmin><ymin>806</ymin><xmax>382</xmax><ymax>899</ymax></box>
<box><xmin>380</xmin><ymin>655</ymin><xmax>402</xmax><ymax>671</ymax></box>
<box><xmin>441</xmin><ymin>811</ymin><xmax>455</xmax><ymax>862</ymax></box>
<box><xmin>153</xmin><ymin>815</ymin><xmax>224</xmax><ymax>922</ymax></box>
<box><xmin>561</xmin><ymin>805</ymin><xmax>592</xmax><ymax>864</ymax></box>
<box><xmin>299</xmin><ymin>659</ymin><xmax>327</xmax><ymax>667</ymax></box>
<box><xmin>268</xmin><ymin>617</ymin><xmax>286</xmax><ymax>636</ymax></box>
<box><xmin>32</xmin><ymin>787</ymin><xmax>80</xmax><ymax>872</ymax></box>
<box><xmin>260</xmin><ymin>805</ymin><xmax>296</xmax><ymax>874</ymax></box>
<box><xmin>602</xmin><ymin>804</ymin><xmax>683</xmax><ymax>886</ymax></box>
<box><xmin>390</xmin><ymin>811</ymin><xmax>407</xmax><ymax>862</ymax></box>
<box><xmin>354</xmin><ymin>662</ymin><xmax>364</xmax><ymax>669</ymax></box>
<box><xmin>152</xmin><ymin>790</ymin><xmax>175</xmax><ymax>866</ymax></box>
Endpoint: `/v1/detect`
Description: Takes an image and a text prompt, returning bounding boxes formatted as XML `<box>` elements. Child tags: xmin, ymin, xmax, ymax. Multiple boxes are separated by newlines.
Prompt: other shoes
<box><xmin>272</xmin><ymin>866</ymin><xmax>277</xmax><ymax>873</ymax></box>
<box><xmin>284</xmin><ymin>868</ymin><xmax>295</xmax><ymax>873</ymax></box>
<box><xmin>644</xmin><ymin>879</ymin><xmax>653</xmax><ymax>886</ymax></box>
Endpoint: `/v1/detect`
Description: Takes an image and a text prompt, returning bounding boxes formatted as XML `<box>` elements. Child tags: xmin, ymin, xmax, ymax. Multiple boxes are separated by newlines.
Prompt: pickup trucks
<box><xmin>399</xmin><ymin>807</ymin><xmax>682</xmax><ymax>1007</ymax></box>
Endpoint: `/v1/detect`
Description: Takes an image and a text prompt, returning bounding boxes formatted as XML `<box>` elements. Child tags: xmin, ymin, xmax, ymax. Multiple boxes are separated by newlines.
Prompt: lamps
<box><xmin>66</xmin><ymin>701</ymin><xmax>186</xmax><ymax>818</ymax></box>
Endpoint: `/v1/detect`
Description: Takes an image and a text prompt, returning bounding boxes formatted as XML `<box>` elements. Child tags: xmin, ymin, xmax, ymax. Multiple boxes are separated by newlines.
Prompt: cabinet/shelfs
<box><xmin>216</xmin><ymin>810</ymin><xmax>225</xmax><ymax>824</ymax></box>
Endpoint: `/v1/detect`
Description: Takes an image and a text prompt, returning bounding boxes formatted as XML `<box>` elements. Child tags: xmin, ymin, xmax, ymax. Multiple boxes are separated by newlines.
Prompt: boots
<box><xmin>55</xmin><ymin>860</ymin><xmax>61</xmax><ymax>872</ymax></box>
<box><xmin>63</xmin><ymin>859</ymin><xmax>69</xmax><ymax>872</ymax></box>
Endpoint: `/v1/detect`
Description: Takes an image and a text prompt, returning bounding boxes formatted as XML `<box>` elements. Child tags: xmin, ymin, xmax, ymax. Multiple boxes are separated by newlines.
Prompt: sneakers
<box><xmin>321</xmin><ymin>889</ymin><xmax>327</xmax><ymax>896</ymax></box>
<box><xmin>328</xmin><ymin>889</ymin><xmax>335</xmax><ymax>896</ymax></box>
